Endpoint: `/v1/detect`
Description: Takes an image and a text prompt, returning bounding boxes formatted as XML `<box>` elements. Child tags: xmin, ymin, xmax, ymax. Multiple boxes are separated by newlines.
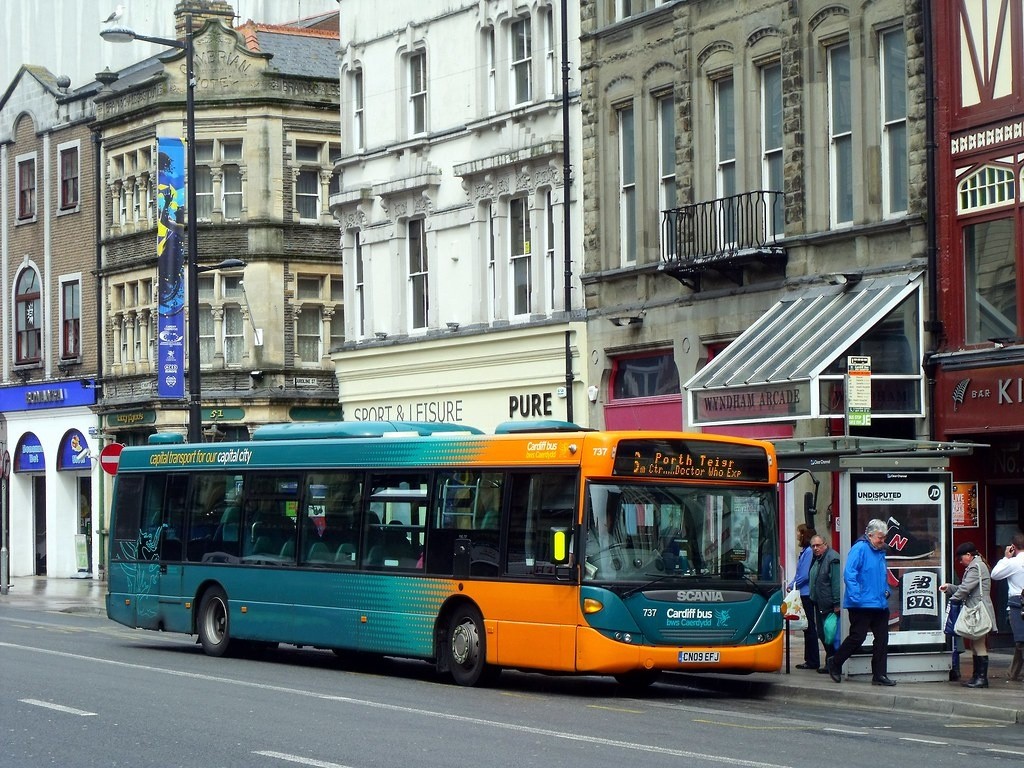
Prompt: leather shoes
<box><xmin>827</xmin><ymin>656</ymin><xmax>841</xmax><ymax>683</ymax></box>
<box><xmin>872</xmin><ymin>674</ymin><xmax>897</xmax><ymax>685</ymax></box>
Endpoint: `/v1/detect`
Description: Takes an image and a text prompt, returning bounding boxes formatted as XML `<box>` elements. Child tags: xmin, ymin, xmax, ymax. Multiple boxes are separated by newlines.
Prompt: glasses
<box><xmin>811</xmin><ymin>543</ymin><xmax>826</xmax><ymax>548</ymax></box>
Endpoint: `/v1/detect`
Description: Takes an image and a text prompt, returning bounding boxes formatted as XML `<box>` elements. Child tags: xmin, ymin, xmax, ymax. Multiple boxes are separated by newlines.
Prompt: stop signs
<box><xmin>100</xmin><ymin>442</ymin><xmax>125</xmax><ymax>476</ymax></box>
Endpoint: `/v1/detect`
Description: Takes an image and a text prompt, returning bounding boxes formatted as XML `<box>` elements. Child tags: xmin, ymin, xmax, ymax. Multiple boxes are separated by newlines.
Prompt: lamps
<box><xmin>375</xmin><ymin>332</ymin><xmax>388</xmax><ymax>339</ymax></box>
<box><xmin>250</xmin><ymin>370</ymin><xmax>263</xmax><ymax>384</ymax></box>
<box><xmin>79</xmin><ymin>379</ymin><xmax>102</xmax><ymax>389</ymax></box>
<box><xmin>446</xmin><ymin>322</ymin><xmax>459</xmax><ymax>332</ymax></box>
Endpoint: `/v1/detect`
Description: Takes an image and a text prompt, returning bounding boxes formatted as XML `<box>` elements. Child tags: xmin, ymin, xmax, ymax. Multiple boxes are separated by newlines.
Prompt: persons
<box><xmin>569</xmin><ymin>512</ymin><xmax>619</xmax><ymax>568</ymax></box>
<box><xmin>705</xmin><ymin>539</ymin><xmax>717</xmax><ymax>573</ymax></box>
<box><xmin>989</xmin><ymin>534</ymin><xmax>1024</xmax><ymax>680</ymax></box>
<box><xmin>386</xmin><ymin>521</ymin><xmax>420</xmax><ymax>560</ymax></box>
<box><xmin>541</xmin><ymin>469</ymin><xmax>570</xmax><ymax>509</ymax></box>
<box><xmin>321</xmin><ymin>520</ymin><xmax>354</xmax><ymax>543</ymax></box>
<box><xmin>938</xmin><ymin>540</ymin><xmax>998</xmax><ymax>689</ymax></box>
<box><xmin>808</xmin><ymin>533</ymin><xmax>842</xmax><ymax>673</ymax></box>
<box><xmin>825</xmin><ymin>518</ymin><xmax>900</xmax><ymax>686</ymax></box>
<box><xmin>787</xmin><ymin>522</ymin><xmax>816</xmax><ymax>670</ymax></box>
<box><xmin>172</xmin><ymin>491</ymin><xmax>186</xmax><ymax>511</ymax></box>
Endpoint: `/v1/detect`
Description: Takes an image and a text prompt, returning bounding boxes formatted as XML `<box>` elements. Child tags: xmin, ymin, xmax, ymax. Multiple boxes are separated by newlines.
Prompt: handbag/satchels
<box><xmin>943</xmin><ymin>599</ymin><xmax>962</xmax><ymax>635</ymax></box>
<box><xmin>953</xmin><ymin>601</ymin><xmax>992</xmax><ymax>639</ymax></box>
<box><xmin>823</xmin><ymin>612</ymin><xmax>837</xmax><ymax>645</ymax></box>
<box><xmin>783</xmin><ymin>582</ymin><xmax>808</xmax><ymax>631</ymax></box>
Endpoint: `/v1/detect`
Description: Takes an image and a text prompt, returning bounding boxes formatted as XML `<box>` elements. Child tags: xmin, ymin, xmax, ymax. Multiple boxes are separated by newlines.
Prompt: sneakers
<box><xmin>882</xmin><ymin>517</ymin><xmax>937</xmax><ymax>560</ymax></box>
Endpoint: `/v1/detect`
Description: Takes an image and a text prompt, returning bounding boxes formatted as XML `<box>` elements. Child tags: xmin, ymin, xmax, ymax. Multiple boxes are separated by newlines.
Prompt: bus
<box><xmin>106</xmin><ymin>419</ymin><xmax>822</xmax><ymax>690</ymax></box>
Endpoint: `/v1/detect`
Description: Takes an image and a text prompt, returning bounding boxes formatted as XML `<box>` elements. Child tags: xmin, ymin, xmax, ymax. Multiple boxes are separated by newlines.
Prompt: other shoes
<box><xmin>795</xmin><ymin>662</ymin><xmax>814</xmax><ymax>669</ymax></box>
<box><xmin>817</xmin><ymin>666</ymin><xmax>828</xmax><ymax>674</ymax></box>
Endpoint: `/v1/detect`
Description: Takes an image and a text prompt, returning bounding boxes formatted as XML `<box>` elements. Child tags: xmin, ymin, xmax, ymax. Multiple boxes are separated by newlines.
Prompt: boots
<box><xmin>961</xmin><ymin>655</ymin><xmax>989</xmax><ymax>688</ymax></box>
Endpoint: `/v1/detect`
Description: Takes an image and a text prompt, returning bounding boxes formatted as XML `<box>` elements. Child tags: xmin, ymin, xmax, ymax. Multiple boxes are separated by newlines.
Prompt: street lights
<box><xmin>99</xmin><ymin>15</ymin><xmax>248</xmax><ymax>445</ymax></box>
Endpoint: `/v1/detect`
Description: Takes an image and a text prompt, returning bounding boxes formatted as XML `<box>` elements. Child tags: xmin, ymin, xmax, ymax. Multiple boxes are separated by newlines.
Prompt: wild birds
<box><xmin>102</xmin><ymin>5</ymin><xmax>126</xmax><ymax>24</ymax></box>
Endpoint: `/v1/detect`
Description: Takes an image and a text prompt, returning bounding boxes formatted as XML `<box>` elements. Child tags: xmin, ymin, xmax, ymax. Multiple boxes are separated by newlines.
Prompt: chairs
<box><xmin>294</xmin><ymin>518</ymin><xmax>320</xmax><ymax>541</ymax></box>
<box><xmin>279</xmin><ymin>538</ymin><xmax>296</xmax><ymax>562</ymax></box>
<box><xmin>353</xmin><ymin>511</ymin><xmax>383</xmax><ymax>544</ymax></box>
<box><xmin>383</xmin><ymin>519</ymin><xmax>411</xmax><ymax>555</ymax></box>
<box><xmin>212</xmin><ymin>507</ymin><xmax>241</xmax><ymax>542</ymax></box>
<box><xmin>366</xmin><ymin>545</ymin><xmax>388</xmax><ymax>564</ymax></box>
<box><xmin>308</xmin><ymin>543</ymin><xmax>333</xmax><ymax>562</ymax></box>
<box><xmin>334</xmin><ymin>543</ymin><xmax>358</xmax><ymax>563</ymax></box>
<box><xmin>320</xmin><ymin>511</ymin><xmax>350</xmax><ymax>546</ymax></box>
<box><xmin>144</xmin><ymin>506</ymin><xmax>219</xmax><ymax>540</ymax></box>
<box><xmin>251</xmin><ymin>536</ymin><xmax>277</xmax><ymax>556</ymax></box>
